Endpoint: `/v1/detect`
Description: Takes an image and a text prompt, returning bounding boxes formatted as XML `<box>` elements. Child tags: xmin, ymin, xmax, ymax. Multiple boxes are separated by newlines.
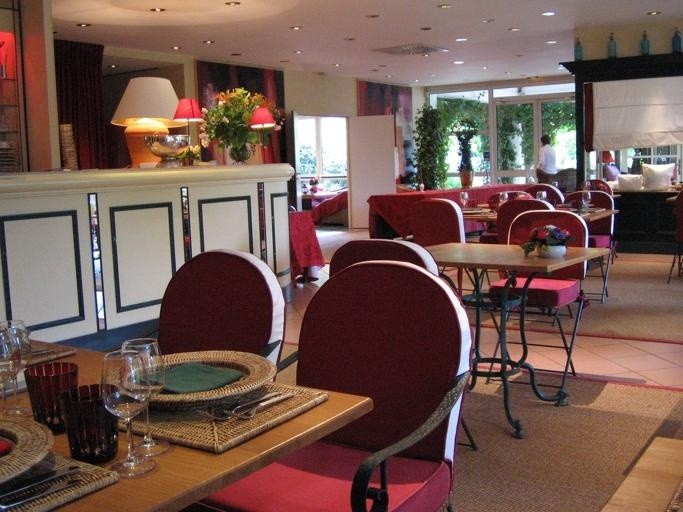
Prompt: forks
<box><xmin>196</xmin><ymin>390</ymin><xmax>281</xmax><ymax>422</ymax></box>
<box><xmin>223</xmin><ymin>390</ymin><xmax>296</xmax><ymax>420</ymax></box>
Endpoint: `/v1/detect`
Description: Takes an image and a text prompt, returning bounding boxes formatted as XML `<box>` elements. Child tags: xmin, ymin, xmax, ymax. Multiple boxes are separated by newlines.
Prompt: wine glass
<box><xmin>551</xmin><ymin>181</ymin><xmax>559</xmax><ymax>186</ymax></box>
<box><xmin>1</xmin><ymin>319</ymin><xmax>33</xmax><ymax>418</ymax></box>
<box><xmin>122</xmin><ymin>337</ymin><xmax>163</xmax><ymax>458</ymax></box>
<box><xmin>536</xmin><ymin>190</ymin><xmax>547</xmax><ymax>202</ymax></box>
<box><xmin>582</xmin><ymin>192</ymin><xmax>592</xmax><ymax>215</ymax></box>
<box><xmin>459</xmin><ymin>191</ymin><xmax>468</xmax><ymax>208</ymax></box>
<box><xmin>0</xmin><ymin>329</ymin><xmax>21</xmax><ymax>421</ymax></box>
<box><xmin>99</xmin><ymin>349</ymin><xmax>157</xmax><ymax>477</ymax></box>
<box><xmin>580</xmin><ymin>181</ymin><xmax>591</xmax><ymax>190</ymax></box>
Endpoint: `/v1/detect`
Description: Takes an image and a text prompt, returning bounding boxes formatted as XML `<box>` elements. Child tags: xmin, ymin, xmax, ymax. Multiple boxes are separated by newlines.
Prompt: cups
<box><xmin>23</xmin><ymin>361</ymin><xmax>79</xmax><ymax>435</ymax></box>
<box><xmin>57</xmin><ymin>383</ymin><xmax>116</xmax><ymax>465</ymax></box>
<box><xmin>499</xmin><ymin>191</ymin><xmax>508</xmax><ymax>204</ymax></box>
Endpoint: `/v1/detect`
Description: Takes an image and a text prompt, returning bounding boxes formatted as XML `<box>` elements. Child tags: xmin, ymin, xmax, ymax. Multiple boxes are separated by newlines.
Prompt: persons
<box><xmin>536</xmin><ymin>135</ymin><xmax>557</xmax><ymax>185</ymax></box>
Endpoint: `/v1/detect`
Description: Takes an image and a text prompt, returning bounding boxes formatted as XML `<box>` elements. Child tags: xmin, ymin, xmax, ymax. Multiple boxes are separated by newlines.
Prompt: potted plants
<box><xmin>413</xmin><ymin>104</ymin><xmax>478</xmax><ymax>191</ymax></box>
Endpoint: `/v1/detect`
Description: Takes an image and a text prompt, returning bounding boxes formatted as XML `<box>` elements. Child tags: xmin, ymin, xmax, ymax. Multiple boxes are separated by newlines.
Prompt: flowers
<box><xmin>198</xmin><ymin>87</ymin><xmax>258</xmax><ymax>153</ymax></box>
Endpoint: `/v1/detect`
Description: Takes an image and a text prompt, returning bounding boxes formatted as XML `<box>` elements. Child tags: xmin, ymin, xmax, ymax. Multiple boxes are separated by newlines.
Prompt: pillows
<box><xmin>607</xmin><ymin>163</ymin><xmax>676</xmax><ymax>192</ymax></box>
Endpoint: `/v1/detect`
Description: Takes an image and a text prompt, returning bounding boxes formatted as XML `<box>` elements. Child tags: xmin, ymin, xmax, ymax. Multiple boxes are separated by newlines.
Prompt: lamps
<box><xmin>109</xmin><ymin>77</ymin><xmax>205</xmax><ymax>169</ymax></box>
<box><xmin>597</xmin><ymin>151</ymin><xmax>616</xmax><ymax>178</ymax></box>
<box><xmin>249</xmin><ymin>107</ymin><xmax>277</xmax><ymax>164</ymax></box>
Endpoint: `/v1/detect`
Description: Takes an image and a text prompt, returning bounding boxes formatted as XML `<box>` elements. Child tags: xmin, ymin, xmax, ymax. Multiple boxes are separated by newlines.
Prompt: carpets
<box><xmin>436</xmin><ymin>251</ymin><xmax>682</xmax><ymax>344</ymax></box>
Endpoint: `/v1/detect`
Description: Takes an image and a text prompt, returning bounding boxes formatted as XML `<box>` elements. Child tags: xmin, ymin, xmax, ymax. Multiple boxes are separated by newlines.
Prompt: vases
<box><xmin>227</xmin><ymin>144</ymin><xmax>256</xmax><ymax>167</ymax></box>
